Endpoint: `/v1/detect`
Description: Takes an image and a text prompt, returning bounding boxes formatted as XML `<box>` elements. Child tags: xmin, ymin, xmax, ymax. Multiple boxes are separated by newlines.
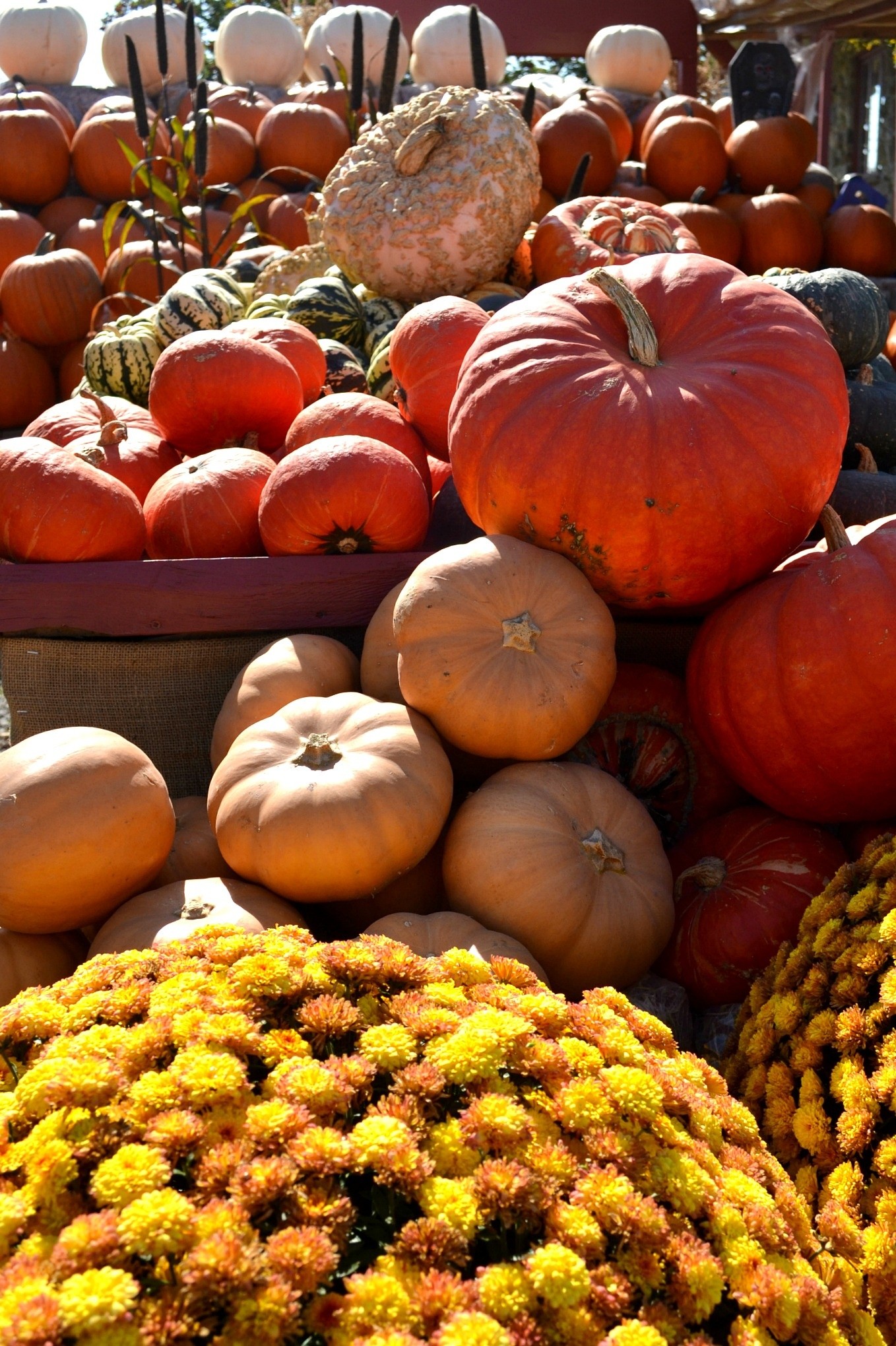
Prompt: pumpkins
<box><xmin>0</xmin><ymin>1</ymin><xmax>896</xmax><ymax>1024</ymax></box>
